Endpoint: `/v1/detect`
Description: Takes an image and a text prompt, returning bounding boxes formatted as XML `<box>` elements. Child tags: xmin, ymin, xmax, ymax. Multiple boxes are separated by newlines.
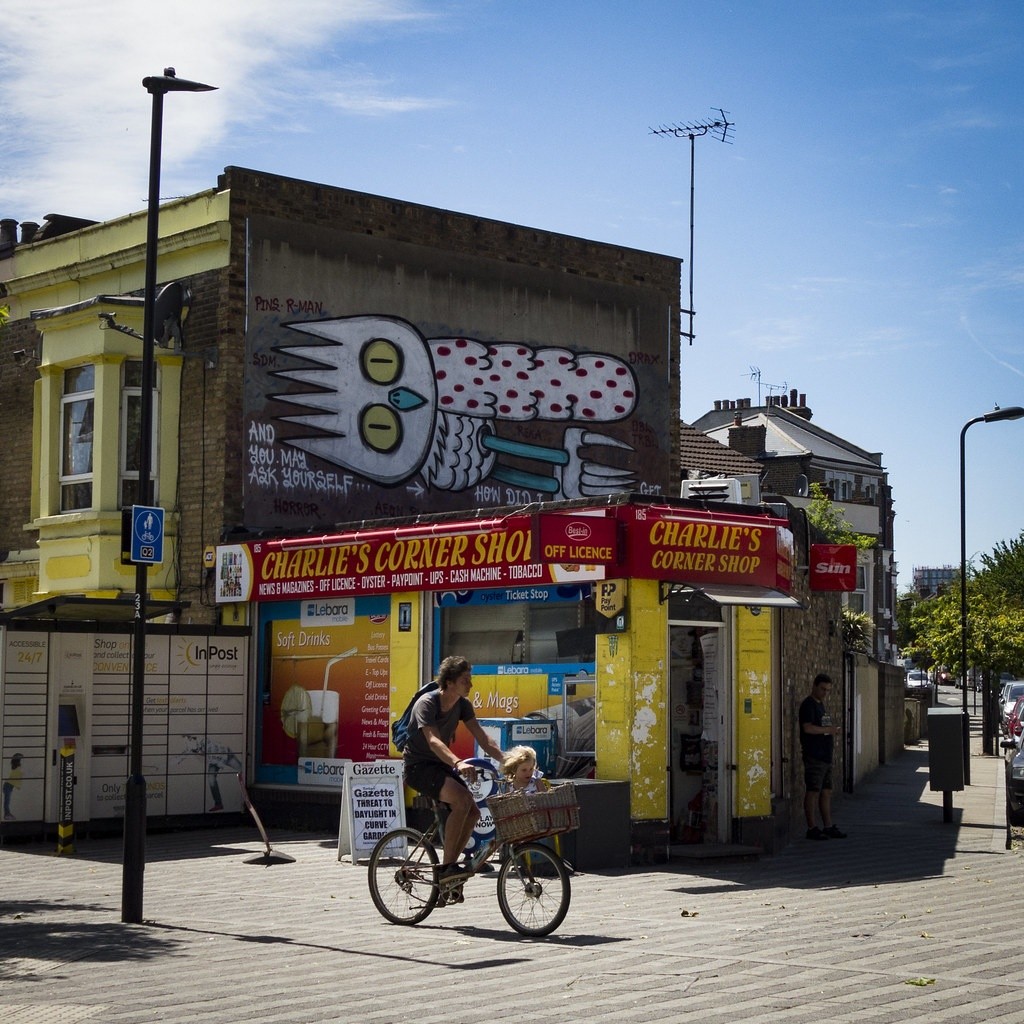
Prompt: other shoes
<box><xmin>807</xmin><ymin>827</ymin><xmax>829</xmax><ymax>840</ymax></box>
<box><xmin>823</xmin><ymin>824</ymin><xmax>847</xmax><ymax>838</ymax></box>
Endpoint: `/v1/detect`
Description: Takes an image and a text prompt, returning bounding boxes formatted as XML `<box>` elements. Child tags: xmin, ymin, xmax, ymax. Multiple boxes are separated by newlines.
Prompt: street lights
<box><xmin>959</xmin><ymin>406</ymin><xmax>1024</xmax><ymax>785</ymax></box>
<box><xmin>115</xmin><ymin>64</ymin><xmax>217</xmax><ymax>923</ymax></box>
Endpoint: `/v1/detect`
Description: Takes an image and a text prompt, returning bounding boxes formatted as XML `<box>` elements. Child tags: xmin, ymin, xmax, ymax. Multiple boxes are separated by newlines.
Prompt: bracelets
<box><xmin>452</xmin><ymin>760</ymin><xmax>464</xmax><ymax>772</ymax></box>
<box><xmin>824</xmin><ymin>727</ymin><xmax>826</xmax><ymax>734</ymax></box>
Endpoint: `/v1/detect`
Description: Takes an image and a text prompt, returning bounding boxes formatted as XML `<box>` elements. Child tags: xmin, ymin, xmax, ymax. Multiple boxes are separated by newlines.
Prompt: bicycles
<box><xmin>367</xmin><ymin>757</ymin><xmax>583</xmax><ymax>938</ymax></box>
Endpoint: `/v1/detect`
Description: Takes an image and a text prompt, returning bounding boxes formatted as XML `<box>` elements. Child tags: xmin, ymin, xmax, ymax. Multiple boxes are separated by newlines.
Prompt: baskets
<box><xmin>487</xmin><ymin>781</ymin><xmax>580</xmax><ymax>842</ymax></box>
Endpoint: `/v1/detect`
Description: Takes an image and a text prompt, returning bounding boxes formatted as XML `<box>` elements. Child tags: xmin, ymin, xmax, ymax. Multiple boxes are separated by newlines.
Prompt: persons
<box><xmin>989</xmin><ymin>689</ymin><xmax>1005</xmax><ymax>730</ymax></box>
<box><xmin>402</xmin><ymin>655</ymin><xmax>514</xmax><ymax>903</ymax></box>
<box><xmin>496</xmin><ymin>744</ymin><xmax>548</xmax><ymax>796</ymax></box>
<box><xmin>798</xmin><ymin>673</ymin><xmax>849</xmax><ymax>841</ymax></box>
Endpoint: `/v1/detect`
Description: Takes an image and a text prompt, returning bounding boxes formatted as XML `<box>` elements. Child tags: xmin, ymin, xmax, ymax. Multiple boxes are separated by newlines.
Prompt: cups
<box><xmin>295</xmin><ymin>691</ymin><xmax>339</xmax><ymax>783</ymax></box>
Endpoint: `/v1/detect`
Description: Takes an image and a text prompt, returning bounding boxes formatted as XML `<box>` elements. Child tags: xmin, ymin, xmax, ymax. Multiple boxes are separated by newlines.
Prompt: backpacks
<box><xmin>391</xmin><ymin>681</ymin><xmax>469</xmax><ymax>752</ymax></box>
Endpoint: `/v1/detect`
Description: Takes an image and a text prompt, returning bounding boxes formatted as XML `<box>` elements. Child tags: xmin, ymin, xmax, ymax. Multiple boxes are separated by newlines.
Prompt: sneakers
<box><xmin>450</xmin><ymin>892</ymin><xmax>465</xmax><ymax>903</ymax></box>
<box><xmin>438</xmin><ymin>863</ymin><xmax>470</xmax><ymax>884</ymax></box>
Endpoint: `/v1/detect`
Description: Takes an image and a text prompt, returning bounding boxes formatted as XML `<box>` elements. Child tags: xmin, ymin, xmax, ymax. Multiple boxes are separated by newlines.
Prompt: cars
<box><xmin>903</xmin><ymin>669</ymin><xmax>932</xmax><ymax>688</ymax></box>
<box><xmin>928</xmin><ymin>664</ymin><xmax>1024</xmax><ymax>754</ymax></box>
<box><xmin>1000</xmin><ymin>724</ymin><xmax>1024</xmax><ymax>825</ymax></box>
<box><xmin>897</xmin><ymin>658</ymin><xmax>915</xmax><ymax>670</ymax></box>
